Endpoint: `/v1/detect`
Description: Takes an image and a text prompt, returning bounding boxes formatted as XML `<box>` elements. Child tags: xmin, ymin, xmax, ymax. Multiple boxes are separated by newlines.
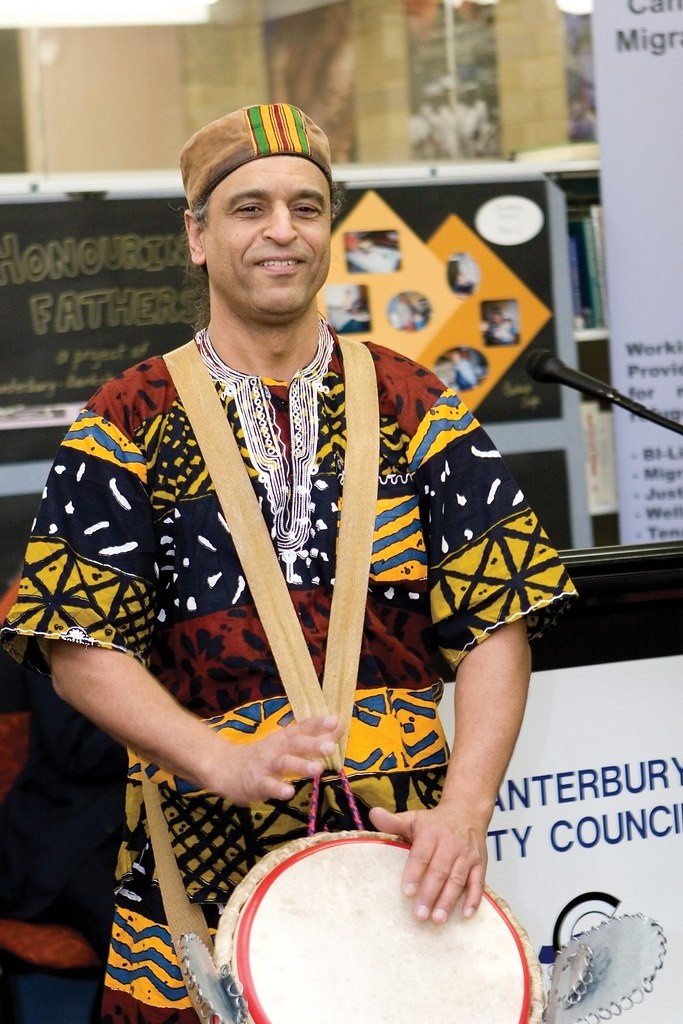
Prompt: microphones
<box><xmin>525</xmin><ymin>349</ymin><xmax>617</xmax><ymax>402</ymax></box>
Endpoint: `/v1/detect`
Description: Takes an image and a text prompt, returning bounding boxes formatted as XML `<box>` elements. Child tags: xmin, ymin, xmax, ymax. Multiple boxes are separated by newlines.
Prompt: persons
<box><xmin>0</xmin><ymin>103</ymin><xmax>579</xmax><ymax>1024</ymax></box>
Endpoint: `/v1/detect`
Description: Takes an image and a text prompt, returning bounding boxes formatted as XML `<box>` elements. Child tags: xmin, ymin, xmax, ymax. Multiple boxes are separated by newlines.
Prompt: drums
<box><xmin>219</xmin><ymin>825</ymin><xmax>548</xmax><ymax>1024</ymax></box>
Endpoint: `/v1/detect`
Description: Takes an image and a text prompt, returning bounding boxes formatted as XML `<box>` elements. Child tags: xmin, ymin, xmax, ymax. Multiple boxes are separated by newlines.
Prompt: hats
<box><xmin>179</xmin><ymin>103</ymin><xmax>333</xmax><ymax>210</ymax></box>
